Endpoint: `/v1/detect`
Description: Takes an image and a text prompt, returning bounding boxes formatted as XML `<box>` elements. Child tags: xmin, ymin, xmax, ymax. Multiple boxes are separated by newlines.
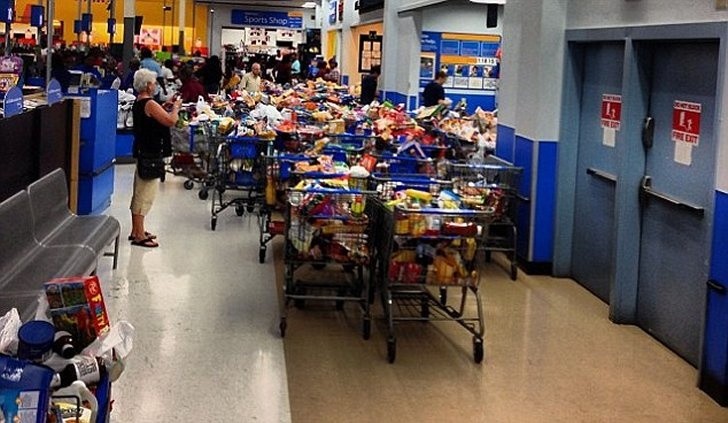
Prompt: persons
<box><xmin>0</xmin><ymin>31</ymin><xmax>340</xmax><ymax>157</ymax></box>
<box><xmin>128</xmin><ymin>68</ymin><xmax>183</xmax><ymax>247</ymax></box>
<box><xmin>359</xmin><ymin>62</ymin><xmax>453</xmax><ymax>108</ymax></box>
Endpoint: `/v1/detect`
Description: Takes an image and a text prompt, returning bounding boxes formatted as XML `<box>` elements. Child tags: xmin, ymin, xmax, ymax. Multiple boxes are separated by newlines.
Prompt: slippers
<box><xmin>128</xmin><ymin>232</ymin><xmax>156</xmax><ymax>239</ymax></box>
<box><xmin>131</xmin><ymin>237</ymin><xmax>158</xmax><ymax>248</ymax></box>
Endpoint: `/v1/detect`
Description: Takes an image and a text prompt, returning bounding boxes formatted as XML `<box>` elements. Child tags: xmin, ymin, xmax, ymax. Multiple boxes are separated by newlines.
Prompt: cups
<box><xmin>350</xmin><ymin>175</ymin><xmax>369</xmax><ymax>216</ymax></box>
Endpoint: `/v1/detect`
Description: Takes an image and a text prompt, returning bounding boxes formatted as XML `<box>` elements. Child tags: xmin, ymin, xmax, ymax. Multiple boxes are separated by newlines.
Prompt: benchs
<box><xmin>0</xmin><ymin>168</ymin><xmax>120</xmax><ymax>324</ymax></box>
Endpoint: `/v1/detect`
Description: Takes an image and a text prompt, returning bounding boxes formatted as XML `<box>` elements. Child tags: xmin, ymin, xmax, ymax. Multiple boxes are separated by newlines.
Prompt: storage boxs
<box><xmin>45</xmin><ymin>276</ymin><xmax>110</xmax><ymax>337</ymax></box>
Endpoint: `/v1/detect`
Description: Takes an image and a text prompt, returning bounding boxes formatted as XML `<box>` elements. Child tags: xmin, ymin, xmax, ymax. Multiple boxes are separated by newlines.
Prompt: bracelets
<box><xmin>172</xmin><ymin>104</ymin><xmax>179</xmax><ymax>108</ymax></box>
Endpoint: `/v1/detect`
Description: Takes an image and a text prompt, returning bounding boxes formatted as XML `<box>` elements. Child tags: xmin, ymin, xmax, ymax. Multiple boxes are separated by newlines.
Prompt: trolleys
<box><xmin>167</xmin><ymin>85</ymin><xmax>525</xmax><ymax>364</ymax></box>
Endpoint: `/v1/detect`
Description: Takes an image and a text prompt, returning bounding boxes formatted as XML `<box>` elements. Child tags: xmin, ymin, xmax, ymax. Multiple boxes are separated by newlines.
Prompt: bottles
<box><xmin>48</xmin><ymin>356</ymin><xmax>107</xmax><ymax>391</ymax></box>
<box><xmin>266</xmin><ymin>175</ymin><xmax>277</xmax><ymax>205</ymax></box>
<box><xmin>53</xmin><ymin>330</ymin><xmax>75</xmax><ymax>359</ymax></box>
<box><xmin>425</xmin><ymin>201</ymin><xmax>440</xmax><ymax>237</ymax></box>
<box><xmin>409</xmin><ymin>203</ymin><xmax>426</xmax><ymax>235</ymax></box>
<box><xmin>394</xmin><ymin>205</ymin><xmax>409</xmax><ymax>235</ymax></box>
